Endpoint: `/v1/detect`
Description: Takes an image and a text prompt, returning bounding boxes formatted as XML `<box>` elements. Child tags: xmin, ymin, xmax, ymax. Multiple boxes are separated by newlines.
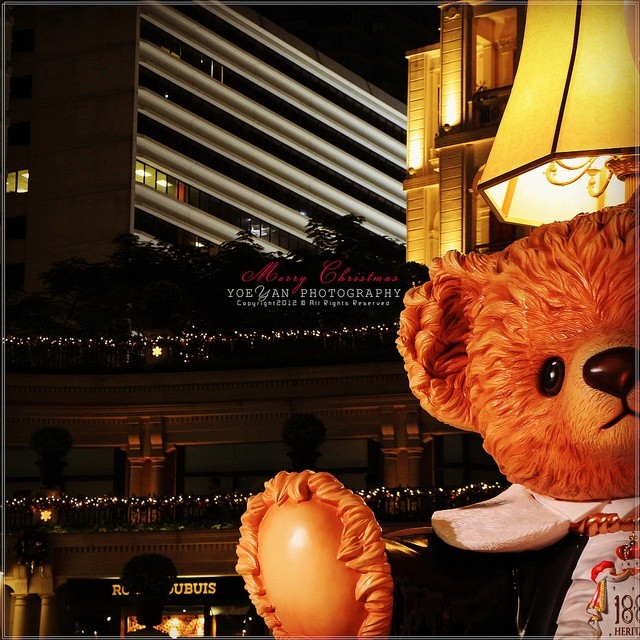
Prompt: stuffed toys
<box><xmin>395</xmin><ymin>206</ymin><xmax>640</xmax><ymax>638</ymax></box>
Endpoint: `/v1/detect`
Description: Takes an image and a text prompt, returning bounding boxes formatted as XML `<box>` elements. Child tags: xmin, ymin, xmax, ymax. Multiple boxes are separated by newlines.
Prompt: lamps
<box><xmin>479</xmin><ymin>0</ymin><xmax>639</xmax><ymax>227</ymax></box>
